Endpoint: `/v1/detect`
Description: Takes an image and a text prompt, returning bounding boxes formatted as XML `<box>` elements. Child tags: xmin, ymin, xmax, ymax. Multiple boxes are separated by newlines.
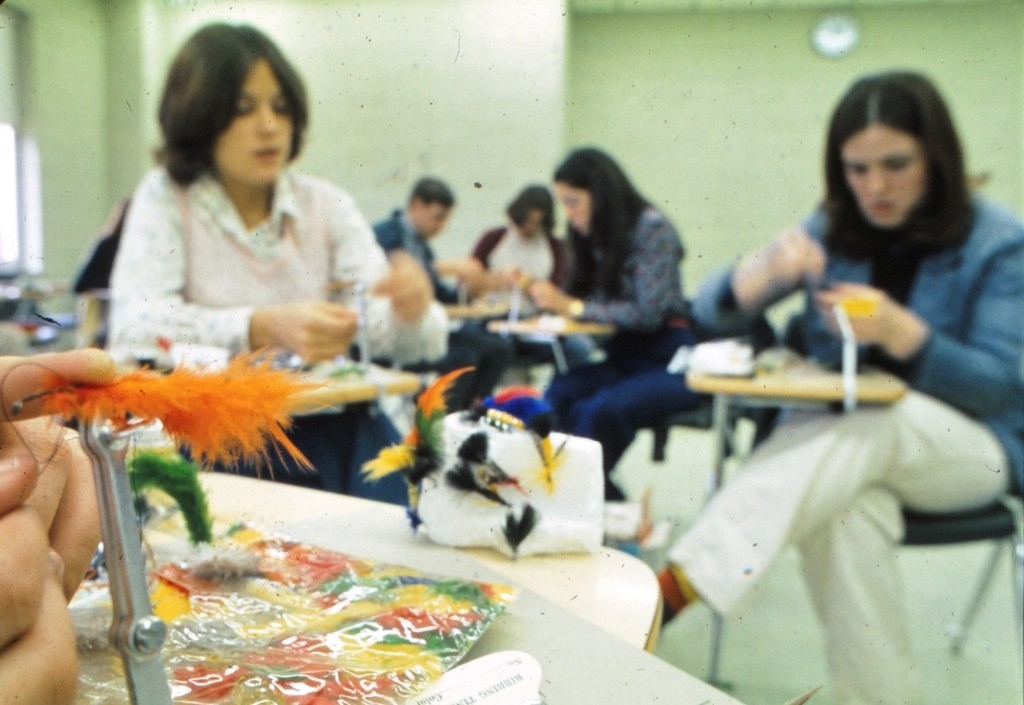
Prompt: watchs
<box><xmin>568</xmin><ymin>299</ymin><xmax>584</xmax><ymax>316</ymax></box>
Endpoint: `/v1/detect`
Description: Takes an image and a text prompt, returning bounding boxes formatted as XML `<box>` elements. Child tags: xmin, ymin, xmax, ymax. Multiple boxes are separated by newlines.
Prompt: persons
<box><xmin>73</xmin><ymin>193</ymin><xmax>127</xmax><ymax>298</ymax></box>
<box><xmin>655</xmin><ymin>68</ymin><xmax>1024</xmax><ymax>705</ymax></box>
<box><xmin>369</xmin><ymin>178</ymin><xmax>509</xmax><ymax>414</ymax></box>
<box><xmin>0</xmin><ymin>348</ymin><xmax>121</xmax><ymax>704</ymax></box>
<box><xmin>456</xmin><ymin>185</ymin><xmax>565</xmax><ymax>407</ymax></box>
<box><xmin>106</xmin><ymin>22</ymin><xmax>452</xmax><ymax>511</ymax></box>
<box><xmin>542</xmin><ymin>147</ymin><xmax>706</xmax><ymax>503</ymax></box>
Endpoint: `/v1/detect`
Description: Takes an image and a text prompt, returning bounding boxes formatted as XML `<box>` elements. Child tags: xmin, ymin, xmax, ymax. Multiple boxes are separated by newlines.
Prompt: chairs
<box><xmin>744</xmin><ymin>406</ymin><xmax>1024</xmax><ymax>705</ymax></box>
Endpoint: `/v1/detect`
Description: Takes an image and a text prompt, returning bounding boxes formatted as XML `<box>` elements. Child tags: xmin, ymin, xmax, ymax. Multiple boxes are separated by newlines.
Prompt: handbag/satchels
<box><xmin>360</xmin><ymin>362</ymin><xmax>610</xmax><ymax>557</ymax></box>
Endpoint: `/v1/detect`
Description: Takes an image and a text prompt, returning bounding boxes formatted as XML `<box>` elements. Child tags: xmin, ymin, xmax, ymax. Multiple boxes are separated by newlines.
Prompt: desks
<box><xmin>274</xmin><ymin>355</ymin><xmax>430</xmax><ymax>415</ymax></box>
<box><xmin>684</xmin><ymin>356</ymin><xmax>907</xmax><ymax>692</ymax></box>
<box><xmin>484</xmin><ymin>314</ymin><xmax>615</xmax><ymax>340</ymax></box>
<box><xmin>149</xmin><ymin>470</ymin><xmax>669</xmax><ymax>705</ymax></box>
